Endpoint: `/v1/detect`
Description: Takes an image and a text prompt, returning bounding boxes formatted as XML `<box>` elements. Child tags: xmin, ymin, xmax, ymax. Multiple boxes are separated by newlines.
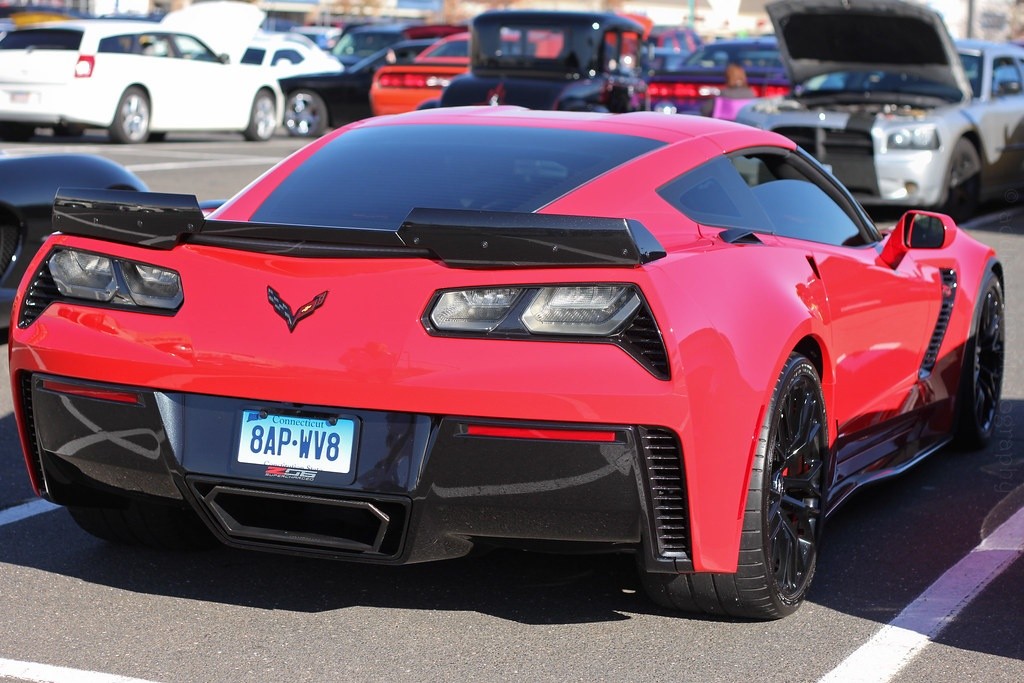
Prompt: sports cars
<box><xmin>5</xmin><ymin>107</ymin><xmax>1008</xmax><ymax>618</ymax></box>
<box><xmin>370</xmin><ymin>14</ymin><xmax>653</xmax><ymax>118</ymax></box>
<box><xmin>0</xmin><ymin>152</ymin><xmax>154</xmax><ymax>339</ymax></box>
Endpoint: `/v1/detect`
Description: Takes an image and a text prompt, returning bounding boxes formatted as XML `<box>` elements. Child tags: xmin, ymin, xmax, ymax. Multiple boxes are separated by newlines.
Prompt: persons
<box><xmin>417</xmin><ymin>73</ymin><xmax>488</xmax><ymax>110</ymax></box>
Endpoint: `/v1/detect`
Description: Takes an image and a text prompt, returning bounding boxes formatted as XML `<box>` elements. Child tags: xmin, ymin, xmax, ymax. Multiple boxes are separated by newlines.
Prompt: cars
<box><xmin>0</xmin><ymin>1</ymin><xmax>284</xmax><ymax>141</ymax></box>
<box><xmin>293</xmin><ymin>28</ymin><xmax>348</xmax><ymax>52</ymax></box>
<box><xmin>418</xmin><ymin>8</ymin><xmax>647</xmax><ymax>115</ymax></box>
<box><xmin>638</xmin><ymin>36</ymin><xmax>851</xmax><ymax>117</ymax></box>
<box><xmin>636</xmin><ymin>23</ymin><xmax>705</xmax><ymax>73</ymax></box>
<box><xmin>3</xmin><ymin>7</ymin><xmax>153</xmax><ymax>53</ymax></box>
<box><xmin>242</xmin><ymin>31</ymin><xmax>344</xmax><ymax>77</ymax></box>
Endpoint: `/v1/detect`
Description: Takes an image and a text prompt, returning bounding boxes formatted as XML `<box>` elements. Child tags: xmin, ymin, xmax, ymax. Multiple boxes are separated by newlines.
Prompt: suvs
<box><xmin>738</xmin><ymin>0</ymin><xmax>1023</xmax><ymax>221</ymax></box>
